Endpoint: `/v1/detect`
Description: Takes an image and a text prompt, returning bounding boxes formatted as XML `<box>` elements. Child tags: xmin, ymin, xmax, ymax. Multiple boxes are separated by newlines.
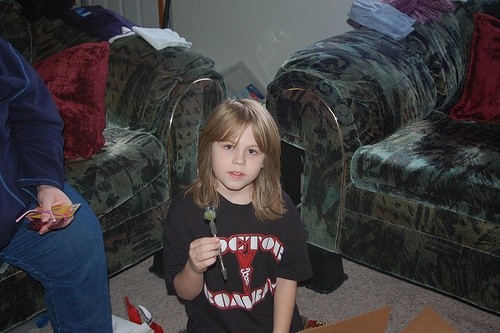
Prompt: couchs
<box><xmin>265</xmin><ymin>0</ymin><xmax>500</xmax><ymax>316</ymax></box>
<box><xmin>0</xmin><ymin>0</ymin><xmax>229</xmax><ymax>332</ymax></box>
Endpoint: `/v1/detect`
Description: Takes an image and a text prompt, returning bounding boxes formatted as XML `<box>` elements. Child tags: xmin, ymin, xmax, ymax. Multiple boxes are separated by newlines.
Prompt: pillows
<box><xmin>29</xmin><ymin>40</ymin><xmax>110</xmax><ymax>164</ymax></box>
<box><xmin>450</xmin><ymin>13</ymin><xmax>500</xmax><ymax>127</ymax></box>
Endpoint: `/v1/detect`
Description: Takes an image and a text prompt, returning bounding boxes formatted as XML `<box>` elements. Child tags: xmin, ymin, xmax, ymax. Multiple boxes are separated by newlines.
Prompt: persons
<box><xmin>163</xmin><ymin>98</ymin><xmax>314</xmax><ymax>333</ymax></box>
<box><xmin>0</xmin><ymin>40</ymin><xmax>114</xmax><ymax>333</ymax></box>
